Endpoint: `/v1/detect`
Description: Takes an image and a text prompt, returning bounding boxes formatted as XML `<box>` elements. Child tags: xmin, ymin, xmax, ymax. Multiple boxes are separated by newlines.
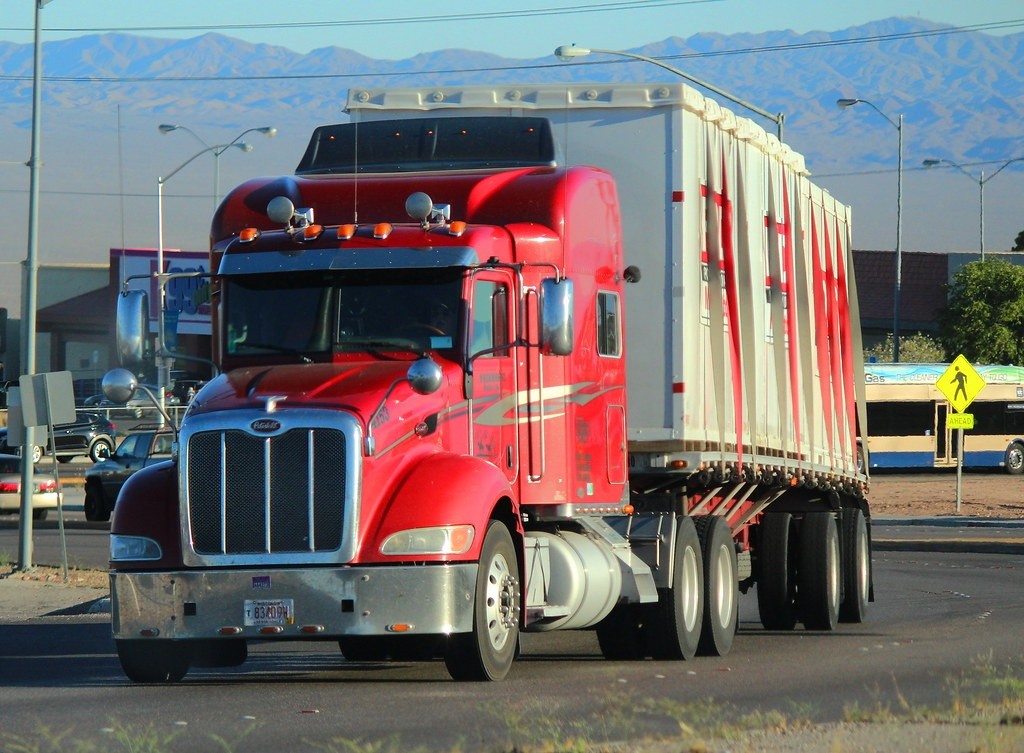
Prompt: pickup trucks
<box><xmin>84</xmin><ymin>425</ymin><xmax>177</xmax><ymax>521</ymax></box>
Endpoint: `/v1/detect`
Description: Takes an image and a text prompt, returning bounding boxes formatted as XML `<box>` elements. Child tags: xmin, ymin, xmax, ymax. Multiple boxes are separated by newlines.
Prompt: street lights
<box><xmin>557</xmin><ymin>44</ymin><xmax>784</xmax><ymax>144</ymax></box>
<box><xmin>922</xmin><ymin>157</ymin><xmax>986</xmax><ymax>262</ymax></box>
<box><xmin>156</xmin><ymin>142</ymin><xmax>251</xmax><ymax>393</ymax></box>
<box><xmin>159</xmin><ymin>122</ymin><xmax>278</xmax><ymax>213</ymax></box>
<box><xmin>835</xmin><ymin>97</ymin><xmax>904</xmax><ymax>360</ymax></box>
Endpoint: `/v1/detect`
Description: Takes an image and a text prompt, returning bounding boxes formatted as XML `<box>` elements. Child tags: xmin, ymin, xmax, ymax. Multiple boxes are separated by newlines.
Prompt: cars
<box><xmin>0</xmin><ymin>455</ymin><xmax>64</xmax><ymax>521</ymax></box>
<box><xmin>85</xmin><ymin>382</ymin><xmax>180</xmax><ymax>418</ymax></box>
<box><xmin>1</xmin><ymin>407</ymin><xmax>115</xmax><ymax>465</ymax></box>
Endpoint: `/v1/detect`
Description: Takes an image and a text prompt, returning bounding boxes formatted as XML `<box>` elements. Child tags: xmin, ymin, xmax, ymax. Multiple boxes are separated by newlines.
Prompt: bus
<box><xmin>853</xmin><ymin>362</ymin><xmax>1024</xmax><ymax>476</ymax></box>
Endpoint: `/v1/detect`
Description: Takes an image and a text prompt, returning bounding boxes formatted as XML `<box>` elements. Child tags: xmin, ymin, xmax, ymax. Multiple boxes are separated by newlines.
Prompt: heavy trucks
<box><xmin>101</xmin><ymin>83</ymin><xmax>873</xmax><ymax>686</ymax></box>
